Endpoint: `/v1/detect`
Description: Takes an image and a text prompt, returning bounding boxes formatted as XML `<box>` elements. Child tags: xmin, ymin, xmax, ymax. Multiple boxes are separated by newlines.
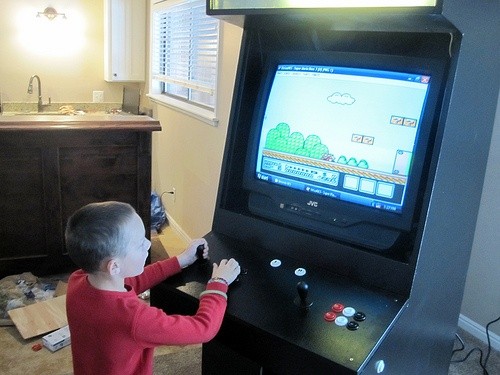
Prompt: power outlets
<box><xmin>93</xmin><ymin>91</ymin><xmax>104</xmax><ymax>103</ymax></box>
<box><xmin>170</xmin><ymin>188</ymin><xmax>176</xmax><ymax>200</ymax></box>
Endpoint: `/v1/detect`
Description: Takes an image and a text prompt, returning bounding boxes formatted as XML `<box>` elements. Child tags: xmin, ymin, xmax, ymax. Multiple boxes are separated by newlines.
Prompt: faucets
<box><xmin>28</xmin><ymin>74</ymin><xmax>44</xmax><ymax>112</ymax></box>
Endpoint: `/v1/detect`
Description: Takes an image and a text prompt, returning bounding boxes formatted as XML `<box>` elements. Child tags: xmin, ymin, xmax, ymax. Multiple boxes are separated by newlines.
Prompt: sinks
<box><xmin>0</xmin><ymin>108</ymin><xmax>82</xmax><ymax>125</ymax></box>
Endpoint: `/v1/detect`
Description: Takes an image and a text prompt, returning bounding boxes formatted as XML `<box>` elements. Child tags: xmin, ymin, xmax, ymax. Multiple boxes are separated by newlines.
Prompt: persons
<box><xmin>65</xmin><ymin>201</ymin><xmax>241</xmax><ymax>375</ymax></box>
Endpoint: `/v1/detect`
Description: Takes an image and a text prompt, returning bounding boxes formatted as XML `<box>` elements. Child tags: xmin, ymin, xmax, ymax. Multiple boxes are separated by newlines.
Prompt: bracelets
<box><xmin>208</xmin><ymin>277</ymin><xmax>229</xmax><ymax>286</ymax></box>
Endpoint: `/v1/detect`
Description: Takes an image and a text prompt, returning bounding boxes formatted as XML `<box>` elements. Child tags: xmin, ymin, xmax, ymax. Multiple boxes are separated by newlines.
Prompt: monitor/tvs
<box><xmin>242</xmin><ymin>49</ymin><xmax>448</xmax><ymax>233</ymax></box>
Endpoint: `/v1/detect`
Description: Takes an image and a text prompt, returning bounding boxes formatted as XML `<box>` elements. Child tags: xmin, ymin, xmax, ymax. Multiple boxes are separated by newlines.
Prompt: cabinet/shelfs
<box><xmin>104</xmin><ymin>0</ymin><xmax>146</xmax><ymax>82</ymax></box>
<box><xmin>0</xmin><ymin>130</ymin><xmax>153</xmax><ymax>278</ymax></box>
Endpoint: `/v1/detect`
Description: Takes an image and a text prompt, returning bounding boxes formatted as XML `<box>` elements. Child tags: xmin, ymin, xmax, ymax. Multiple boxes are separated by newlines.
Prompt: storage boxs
<box><xmin>42</xmin><ymin>325</ymin><xmax>71</xmax><ymax>352</ymax></box>
